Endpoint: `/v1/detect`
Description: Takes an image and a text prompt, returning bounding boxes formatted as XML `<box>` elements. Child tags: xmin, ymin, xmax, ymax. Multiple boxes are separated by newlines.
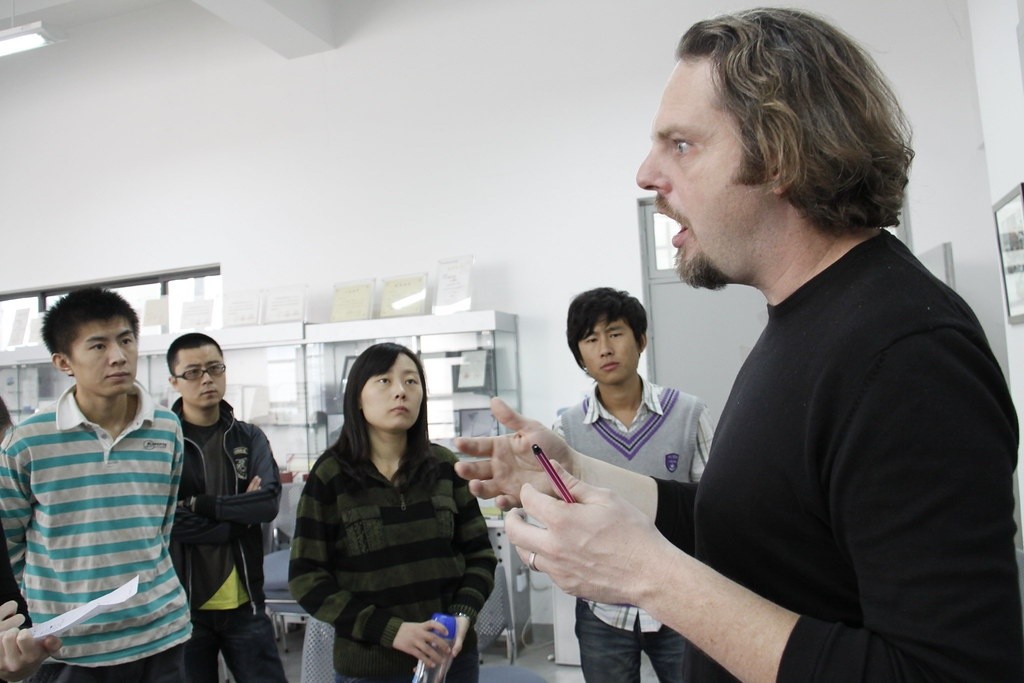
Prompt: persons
<box><xmin>454</xmin><ymin>8</ymin><xmax>1024</xmax><ymax>683</ymax></box>
<box><xmin>288</xmin><ymin>342</ymin><xmax>497</xmax><ymax>683</ymax></box>
<box><xmin>0</xmin><ymin>287</ymin><xmax>286</xmax><ymax>683</ymax></box>
<box><xmin>552</xmin><ymin>288</ymin><xmax>715</xmax><ymax>683</ymax></box>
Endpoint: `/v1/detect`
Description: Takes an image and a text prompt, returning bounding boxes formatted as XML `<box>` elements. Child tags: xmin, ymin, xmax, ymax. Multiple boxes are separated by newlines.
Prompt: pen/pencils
<box><xmin>531</xmin><ymin>443</ymin><xmax>576</xmax><ymax>504</ymax></box>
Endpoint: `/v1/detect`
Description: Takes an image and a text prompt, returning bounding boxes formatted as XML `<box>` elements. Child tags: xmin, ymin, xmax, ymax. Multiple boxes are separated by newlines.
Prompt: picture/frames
<box><xmin>990</xmin><ymin>182</ymin><xmax>1024</xmax><ymax>325</ymax></box>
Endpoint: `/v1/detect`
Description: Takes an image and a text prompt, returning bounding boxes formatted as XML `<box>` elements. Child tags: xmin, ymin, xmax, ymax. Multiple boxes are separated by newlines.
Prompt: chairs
<box><xmin>473</xmin><ymin>565</ymin><xmax>548</xmax><ymax>683</ymax></box>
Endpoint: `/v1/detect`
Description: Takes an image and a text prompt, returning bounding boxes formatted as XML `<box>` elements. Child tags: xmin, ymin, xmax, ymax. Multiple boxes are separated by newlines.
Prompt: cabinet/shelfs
<box><xmin>0</xmin><ymin>312</ymin><xmax>535</xmax><ymax>666</ymax></box>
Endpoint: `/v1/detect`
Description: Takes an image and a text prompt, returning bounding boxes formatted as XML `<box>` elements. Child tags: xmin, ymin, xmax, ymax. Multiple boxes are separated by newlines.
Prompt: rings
<box><xmin>528</xmin><ymin>552</ymin><xmax>542</xmax><ymax>573</ymax></box>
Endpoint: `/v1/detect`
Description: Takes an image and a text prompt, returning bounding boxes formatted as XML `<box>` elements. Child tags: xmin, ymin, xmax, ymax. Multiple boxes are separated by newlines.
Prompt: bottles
<box><xmin>412</xmin><ymin>613</ymin><xmax>456</xmax><ymax>683</ymax></box>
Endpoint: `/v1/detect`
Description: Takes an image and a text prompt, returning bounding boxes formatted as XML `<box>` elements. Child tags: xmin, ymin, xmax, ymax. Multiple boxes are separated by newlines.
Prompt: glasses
<box><xmin>176</xmin><ymin>364</ymin><xmax>226</xmax><ymax>381</ymax></box>
